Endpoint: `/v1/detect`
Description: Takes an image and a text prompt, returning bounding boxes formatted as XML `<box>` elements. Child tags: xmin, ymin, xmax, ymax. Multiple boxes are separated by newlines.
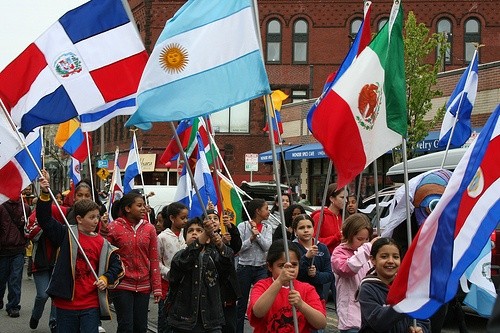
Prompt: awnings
<box><xmin>258</xmin><ymin>145</ymin><xmax>301</xmax><ymax>162</ymax></box>
<box><xmin>286</xmin><ymin>127</ymin><xmax>483</xmax><ymax>160</ymax></box>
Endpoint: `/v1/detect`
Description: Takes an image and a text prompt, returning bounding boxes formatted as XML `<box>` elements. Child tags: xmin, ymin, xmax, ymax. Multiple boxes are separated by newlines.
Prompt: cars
<box><xmin>239</xmin><ymin>179</ymin><xmax>293</xmax><ymax>210</ymax></box>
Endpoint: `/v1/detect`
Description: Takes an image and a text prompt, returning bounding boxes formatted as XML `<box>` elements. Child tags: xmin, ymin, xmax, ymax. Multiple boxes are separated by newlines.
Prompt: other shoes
<box><xmin>30</xmin><ymin>316</ymin><xmax>40</xmax><ymax>329</ymax></box>
<box><xmin>28</xmin><ymin>275</ymin><xmax>33</xmax><ymax>280</ymax></box>
<box><xmin>98</xmin><ymin>325</ymin><xmax>106</xmax><ymax>333</ymax></box>
<box><xmin>8</xmin><ymin>310</ymin><xmax>20</xmax><ymax>317</ymax></box>
<box><xmin>51</xmin><ymin>327</ymin><xmax>57</xmax><ymax>333</ymax></box>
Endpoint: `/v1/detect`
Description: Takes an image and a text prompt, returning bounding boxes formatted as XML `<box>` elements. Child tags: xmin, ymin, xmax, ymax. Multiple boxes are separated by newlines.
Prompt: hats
<box><xmin>201</xmin><ymin>209</ymin><xmax>220</xmax><ymax>221</ymax></box>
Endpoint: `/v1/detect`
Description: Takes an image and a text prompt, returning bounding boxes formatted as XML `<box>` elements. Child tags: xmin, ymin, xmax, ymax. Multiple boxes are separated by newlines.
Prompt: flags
<box><xmin>438</xmin><ymin>50</ymin><xmax>479</xmax><ymax>147</ymax></box>
<box><xmin>387</xmin><ymin>104</ymin><xmax>500</xmax><ymax>320</ymax></box>
<box><xmin>312</xmin><ymin>0</ymin><xmax>406</xmax><ymax>189</ymax></box>
<box><xmin>0</xmin><ymin>126</ymin><xmax>43</xmax><ymax>201</ymax></box>
<box><xmin>67</xmin><ymin>138</ymin><xmax>138</xmax><ymax>204</ymax></box>
<box><xmin>161</xmin><ymin>113</ymin><xmax>242</xmax><ymax>235</ymax></box>
<box><xmin>125</xmin><ymin>0</ymin><xmax>271</xmax><ymax>130</ymax></box>
<box><xmin>306</xmin><ymin>0</ymin><xmax>371</xmax><ymax>133</ymax></box>
<box><xmin>0</xmin><ymin>1</ymin><xmax>147</xmax><ymax>139</ymax></box>
<box><xmin>54</xmin><ymin>118</ymin><xmax>92</xmax><ymax>163</ymax></box>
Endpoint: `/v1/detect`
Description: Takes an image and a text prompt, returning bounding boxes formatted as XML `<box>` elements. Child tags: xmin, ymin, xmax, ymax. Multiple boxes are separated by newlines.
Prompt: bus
<box><xmin>102</xmin><ymin>168</ymin><xmax>222</xmax><ymax>220</ymax></box>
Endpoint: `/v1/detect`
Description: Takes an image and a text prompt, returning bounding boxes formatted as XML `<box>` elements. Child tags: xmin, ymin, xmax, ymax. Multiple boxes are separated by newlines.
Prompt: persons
<box><xmin>311</xmin><ymin>182</ymin><xmax>349</xmax><ymax>255</ymax></box>
<box><xmin>247</xmin><ymin>240</ymin><xmax>326</xmax><ymax>333</ymax></box>
<box><xmin>235</xmin><ymin>198</ymin><xmax>272</xmax><ymax>333</ymax></box>
<box><xmin>352</xmin><ymin>238</ymin><xmax>436</xmax><ymax>333</ymax></box>
<box><xmin>169</xmin><ymin>217</ymin><xmax>235</xmax><ymax>333</ymax></box>
<box><xmin>0</xmin><ymin>180</ymin><xmax>187</xmax><ymax>333</ymax></box>
<box><xmin>202</xmin><ymin>209</ymin><xmax>242</xmax><ymax>333</ymax></box>
<box><xmin>331</xmin><ymin>214</ymin><xmax>381</xmax><ymax>333</ymax></box>
<box><xmin>268</xmin><ymin>193</ymin><xmax>311</xmax><ymax>243</ymax></box>
<box><xmin>291</xmin><ymin>215</ymin><xmax>333</xmax><ymax>301</ymax></box>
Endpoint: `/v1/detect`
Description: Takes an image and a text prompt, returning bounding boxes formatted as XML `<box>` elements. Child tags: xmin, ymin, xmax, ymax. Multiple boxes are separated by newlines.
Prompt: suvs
<box><xmin>361</xmin><ymin>148</ymin><xmax>466</xmax><ymax>229</ymax></box>
